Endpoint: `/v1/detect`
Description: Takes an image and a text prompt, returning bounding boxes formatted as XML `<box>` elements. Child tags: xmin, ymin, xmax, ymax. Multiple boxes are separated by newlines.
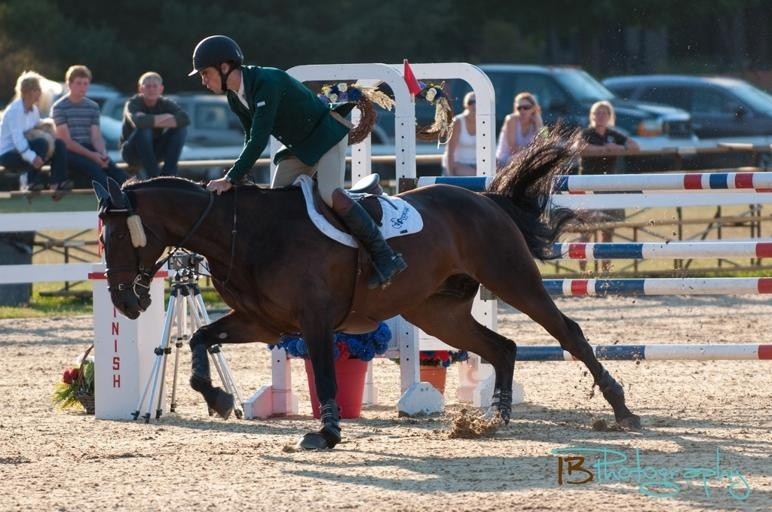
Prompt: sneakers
<box><xmin>52</xmin><ymin>180</ymin><xmax>74</xmax><ymax>201</ymax></box>
<box><xmin>28</xmin><ymin>182</ymin><xmax>49</xmax><ymax>190</ymax></box>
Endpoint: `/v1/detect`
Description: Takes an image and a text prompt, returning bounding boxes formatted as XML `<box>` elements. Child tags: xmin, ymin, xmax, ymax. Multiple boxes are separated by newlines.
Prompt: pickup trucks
<box><xmin>370</xmin><ymin>64</ymin><xmax>697</xmax><ymax>149</ymax></box>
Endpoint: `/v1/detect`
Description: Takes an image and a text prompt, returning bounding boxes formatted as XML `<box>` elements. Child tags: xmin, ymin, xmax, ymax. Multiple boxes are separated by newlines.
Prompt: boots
<box><xmin>343</xmin><ymin>203</ymin><xmax>407</xmax><ymax>289</ymax></box>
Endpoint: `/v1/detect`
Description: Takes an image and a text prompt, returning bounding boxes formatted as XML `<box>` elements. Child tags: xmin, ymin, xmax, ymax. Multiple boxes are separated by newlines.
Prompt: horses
<box><xmin>91</xmin><ymin>117</ymin><xmax>640</xmax><ymax>450</ymax></box>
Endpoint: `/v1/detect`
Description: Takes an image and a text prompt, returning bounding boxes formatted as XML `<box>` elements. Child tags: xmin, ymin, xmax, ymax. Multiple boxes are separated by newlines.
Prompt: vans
<box><xmin>602</xmin><ymin>74</ymin><xmax>772</xmax><ymax>146</ymax></box>
<box><xmin>103</xmin><ymin>96</ymin><xmax>271</xmax><ymax>158</ymax></box>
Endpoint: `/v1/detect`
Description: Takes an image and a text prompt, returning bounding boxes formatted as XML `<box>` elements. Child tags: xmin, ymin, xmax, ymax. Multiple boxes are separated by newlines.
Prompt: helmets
<box><xmin>188</xmin><ymin>35</ymin><xmax>243</xmax><ymax>76</ymax></box>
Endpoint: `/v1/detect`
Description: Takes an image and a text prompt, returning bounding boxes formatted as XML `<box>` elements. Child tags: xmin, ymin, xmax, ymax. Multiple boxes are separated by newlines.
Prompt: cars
<box><xmin>61</xmin><ymin>82</ymin><xmax>186</xmax><ymax>150</ymax></box>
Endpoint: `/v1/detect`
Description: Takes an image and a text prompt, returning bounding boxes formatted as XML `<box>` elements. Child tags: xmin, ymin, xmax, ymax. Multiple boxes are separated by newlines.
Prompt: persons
<box><xmin>439</xmin><ymin>91</ymin><xmax>477</xmax><ymax>176</ymax></box>
<box><xmin>48</xmin><ymin>64</ymin><xmax>131</xmax><ymax>189</ymax></box>
<box><xmin>572</xmin><ymin>100</ymin><xmax>641</xmax><ymax>174</ymax></box>
<box><xmin>0</xmin><ymin>72</ymin><xmax>75</xmax><ymax>192</ymax></box>
<box><xmin>119</xmin><ymin>71</ymin><xmax>191</xmax><ymax>180</ymax></box>
<box><xmin>187</xmin><ymin>33</ymin><xmax>408</xmax><ymax>290</ymax></box>
<box><xmin>495</xmin><ymin>91</ymin><xmax>544</xmax><ymax>172</ymax></box>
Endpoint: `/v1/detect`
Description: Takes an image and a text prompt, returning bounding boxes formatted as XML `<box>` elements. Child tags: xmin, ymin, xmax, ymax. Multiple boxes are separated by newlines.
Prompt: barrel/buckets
<box><xmin>306</xmin><ymin>344</ymin><xmax>370</xmax><ymax>422</ymax></box>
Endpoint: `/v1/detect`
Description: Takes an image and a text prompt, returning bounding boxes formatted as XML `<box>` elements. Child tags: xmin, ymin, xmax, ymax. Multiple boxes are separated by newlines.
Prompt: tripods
<box><xmin>131</xmin><ymin>280</ymin><xmax>245</xmax><ymax>424</ymax></box>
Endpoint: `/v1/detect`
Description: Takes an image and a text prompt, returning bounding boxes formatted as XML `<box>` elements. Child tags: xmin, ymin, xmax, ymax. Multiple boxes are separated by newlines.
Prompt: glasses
<box><xmin>516</xmin><ymin>104</ymin><xmax>532</xmax><ymax>110</ymax></box>
